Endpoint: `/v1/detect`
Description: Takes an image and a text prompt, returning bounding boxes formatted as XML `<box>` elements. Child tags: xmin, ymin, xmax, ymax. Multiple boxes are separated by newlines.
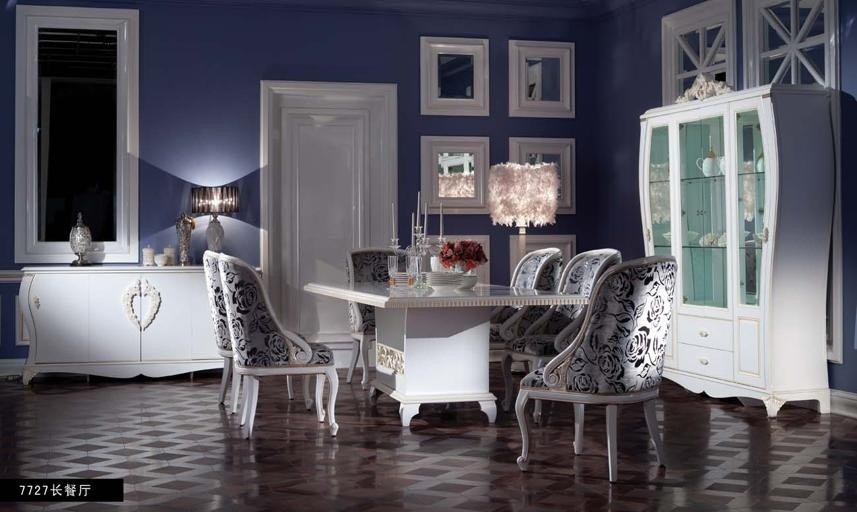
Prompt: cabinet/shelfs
<box><xmin>636</xmin><ymin>82</ymin><xmax>836</xmax><ymax>420</ymax></box>
<box><xmin>16</xmin><ymin>264</ymin><xmax>262</xmax><ymax>389</ymax></box>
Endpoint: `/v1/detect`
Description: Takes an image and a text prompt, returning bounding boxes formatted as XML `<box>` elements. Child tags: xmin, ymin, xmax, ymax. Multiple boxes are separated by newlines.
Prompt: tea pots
<box><xmin>694</xmin><ymin>157</ymin><xmax>723</xmax><ymax>175</ymax></box>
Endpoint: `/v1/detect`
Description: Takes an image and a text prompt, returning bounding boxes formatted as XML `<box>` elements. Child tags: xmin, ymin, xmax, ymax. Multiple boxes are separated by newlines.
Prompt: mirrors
<box><xmin>507</xmin><ymin>136</ymin><xmax>577</xmax><ymax>217</ymax></box>
<box><xmin>420</xmin><ymin>135</ymin><xmax>490</xmax><ymax>215</ymax></box>
<box><xmin>420</xmin><ymin>34</ymin><xmax>491</xmax><ymax>118</ymax></box>
<box><xmin>505</xmin><ymin>39</ymin><xmax>577</xmax><ymax>120</ymax></box>
<box><xmin>14</xmin><ymin>2</ymin><xmax>141</xmax><ymax>264</ymax></box>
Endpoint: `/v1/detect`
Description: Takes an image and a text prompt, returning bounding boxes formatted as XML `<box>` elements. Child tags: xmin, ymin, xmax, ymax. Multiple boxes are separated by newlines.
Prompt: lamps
<box><xmin>489</xmin><ymin>159</ymin><xmax>562</xmax><ymax>261</ymax></box>
<box><xmin>188</xmin><ymin>183</ymin><xmax>241</xmax><ymax>255</ymax></box>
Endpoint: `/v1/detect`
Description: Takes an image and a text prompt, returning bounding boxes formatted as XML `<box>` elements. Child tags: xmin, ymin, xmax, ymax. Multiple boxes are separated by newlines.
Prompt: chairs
<box><xmin>509</xmin><ymin>253</ymin><xmax>679</xmax><ymax>483</ymax></box>
<box><xmin>338</xmin><ymin>245</ymin><xmax>414</xmax><ymax>392</ymax></box>
<box><xmin>488</xmin><ymin>243</ymin><xmax>566</xmax><ymax>376</ymax></box>
<box><xmin>199</xmin><ymin>245</ymin><xmax>314</xmax><ymax>419</ymax></box>
<box><xmin>502</xmin><ymin>247</ymin><xmax>624</xmax><ymax>425</ymax></box>
<box><xmin>215</xmin><ymin>254</ymin><xmax>343</xmax><ymax>439</ymax></box>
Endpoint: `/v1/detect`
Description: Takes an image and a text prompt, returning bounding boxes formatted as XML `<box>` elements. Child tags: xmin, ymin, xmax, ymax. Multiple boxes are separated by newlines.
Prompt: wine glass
<box><xmin>386</xmin><ymin>255</ymin><xmax>398</xmax><ymax>289</ymax></box>
<box><xmin>405</xmin><ymin>256</ymin><xmax>419</xmax><ymax>292</ymax></box>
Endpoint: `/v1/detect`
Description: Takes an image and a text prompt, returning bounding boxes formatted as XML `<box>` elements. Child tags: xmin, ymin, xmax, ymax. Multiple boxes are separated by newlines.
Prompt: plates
<box><xmin>425</xmin><ymin>271</ymin><xmax>464</xmax><ymax>293</ymax></box>
<box><xmin>393</xmin><ymin>272</ymin><xmax>425</xmax><ymax>286</ymax></box>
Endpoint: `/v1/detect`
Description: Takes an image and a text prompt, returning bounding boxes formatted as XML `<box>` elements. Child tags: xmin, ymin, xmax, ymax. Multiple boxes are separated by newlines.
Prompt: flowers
<box><xmin>439</xmin><ymin>240</ymin><xmax>487</xmax><ymax>272</ymax></box>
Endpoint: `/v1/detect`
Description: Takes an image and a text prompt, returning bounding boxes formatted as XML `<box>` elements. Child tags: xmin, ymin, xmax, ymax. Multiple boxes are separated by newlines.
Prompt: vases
<box><xmin>448</xmin><ymin>260</ymin><xmax>478</xmax><ymax>290</ymax></box>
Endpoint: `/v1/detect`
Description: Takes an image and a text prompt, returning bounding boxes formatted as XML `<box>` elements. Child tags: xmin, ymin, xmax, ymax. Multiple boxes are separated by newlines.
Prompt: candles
<box><xmin>411</xmin><ymin>212</ymin><xmax>414</xmax><ymax>245</ymax></box>
<box><xmin>391</xmin><ymin>202</ymin><xmax>395</xmax><ymax>238</ymax></box>
<box><xmin>417</xmin><ymin>190</ymin><xmax>420</xmax><ymax>221</ymax></box>
<box><xmin>424</xmin><ymin>201</ymin><xmax>428</xmax><ymax>235</ymax></box>
<box><xmin>440</xmin><ymin>198</ymin><xmax>443</xmax><ymax>237</ymax></box>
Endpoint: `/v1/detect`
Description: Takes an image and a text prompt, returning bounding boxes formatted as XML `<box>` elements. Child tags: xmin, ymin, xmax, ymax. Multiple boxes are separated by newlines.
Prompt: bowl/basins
<box><xmin>457</xmin><ymin>274</ymin><xmax>478</xmax><ymax>289</ymax></box>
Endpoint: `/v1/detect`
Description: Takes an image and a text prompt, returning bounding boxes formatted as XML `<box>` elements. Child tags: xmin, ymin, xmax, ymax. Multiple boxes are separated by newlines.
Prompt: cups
<box><xmin>142</xmin><ymin>244</ymin><xmax>177</xmax><ymax>266</ymax></box>
